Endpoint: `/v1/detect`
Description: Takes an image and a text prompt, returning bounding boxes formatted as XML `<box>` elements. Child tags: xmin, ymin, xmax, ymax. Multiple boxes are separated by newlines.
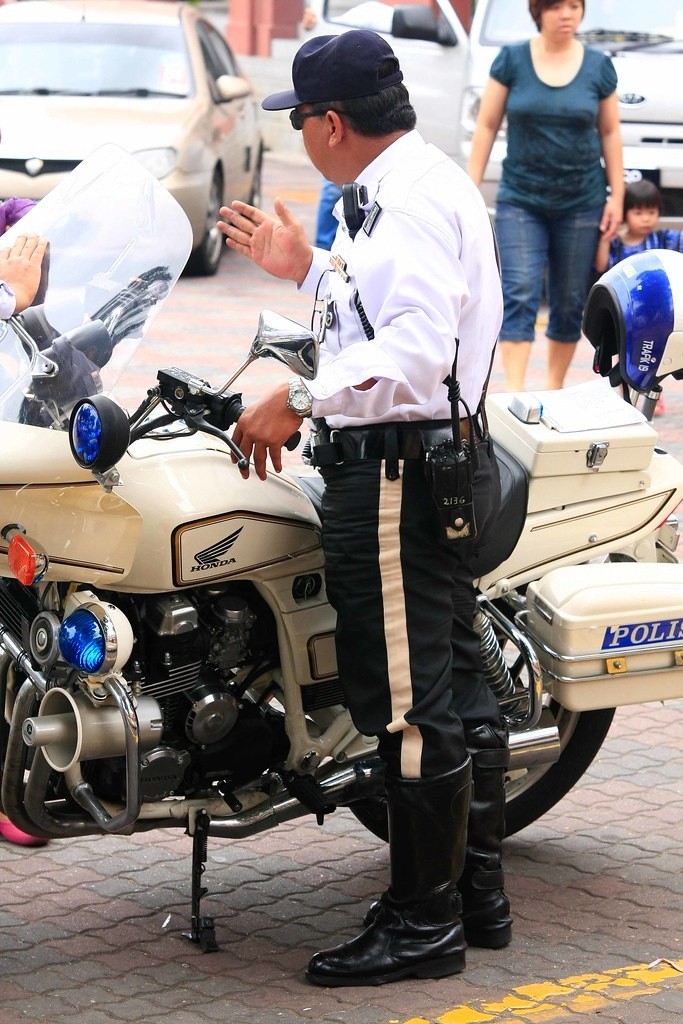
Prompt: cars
<box><xmin>1</xmin><ymin>1</ymin><xmax>269</xmax><ymax>275</ymax></box>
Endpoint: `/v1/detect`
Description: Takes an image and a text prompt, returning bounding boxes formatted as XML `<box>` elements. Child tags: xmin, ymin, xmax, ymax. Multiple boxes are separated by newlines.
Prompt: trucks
<box><xmin>294</xmin><ymin>0</ymin><xmax>682</xmax><ymax>208</ymax></box>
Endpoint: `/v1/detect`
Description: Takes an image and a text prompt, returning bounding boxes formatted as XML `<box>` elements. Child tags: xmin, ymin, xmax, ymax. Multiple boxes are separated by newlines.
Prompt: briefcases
<box><xmin>480</xmin><ymin>386</ymin><xmax>659</xmax><ymax>480</ymax></box>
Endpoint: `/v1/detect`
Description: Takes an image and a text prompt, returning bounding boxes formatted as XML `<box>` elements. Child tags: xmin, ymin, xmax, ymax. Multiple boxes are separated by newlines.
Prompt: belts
<box><xmin>326</xmin><ymin>411</ymin><xmax>482</xmax><ymax>460</ymax></box>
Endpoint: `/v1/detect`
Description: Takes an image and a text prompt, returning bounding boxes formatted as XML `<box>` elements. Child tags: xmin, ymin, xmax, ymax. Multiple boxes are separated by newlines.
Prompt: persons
<box><xmin>467</xmin><ymin>0</ymin><xmax>624</xmax><ymax>392</ymax></box>
<box><xmin>0</xmin><ymin>231</ymin><xmax>49</xmax><ymax>320</ymax></box>
<box><xmin>596</xmin><ymin>179</ymin><xmax>683</xmax><ymax>415</ymax></box>
<box><xmin>216</xmin><ymin>31</ymin><xmax>513</xmax><ymax>986</ymax></box>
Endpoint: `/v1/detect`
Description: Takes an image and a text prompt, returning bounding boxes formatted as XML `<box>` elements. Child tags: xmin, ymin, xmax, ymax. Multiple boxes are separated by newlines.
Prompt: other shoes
<box><xmin>650</xmin><ymin>392</ymin><xmax>668</xmax><ymax>418</ymax></box>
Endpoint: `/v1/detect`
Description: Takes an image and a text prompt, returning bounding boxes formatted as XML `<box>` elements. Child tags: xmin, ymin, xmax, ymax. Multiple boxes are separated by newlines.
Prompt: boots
<box><xmin>363</xmin><ymin>724</ymin><xmax>514</xmax><ymax>949</ymax></box>
<box><xmin>304</xmin><ymin>750</ymin><xmax>474</xmax><ymax>987</ymax></box>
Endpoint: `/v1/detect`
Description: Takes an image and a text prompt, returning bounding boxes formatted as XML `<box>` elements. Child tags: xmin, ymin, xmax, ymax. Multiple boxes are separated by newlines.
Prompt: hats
<box><xmin>261</xmin><ymin>30</ymin><xmax>403</xmax><ymax>111</ymax></box>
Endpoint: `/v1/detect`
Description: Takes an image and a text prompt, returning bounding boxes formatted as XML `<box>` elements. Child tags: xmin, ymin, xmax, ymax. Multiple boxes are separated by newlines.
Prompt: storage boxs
<box><xmin>525</xmin><ymin>562</ymin><xmax>683</xmax><ymax>714</ymax></box>
<box><xmin>481</xmin><ymin>388</ymin><xmax>659</xmax><ymax>478</ymax></box>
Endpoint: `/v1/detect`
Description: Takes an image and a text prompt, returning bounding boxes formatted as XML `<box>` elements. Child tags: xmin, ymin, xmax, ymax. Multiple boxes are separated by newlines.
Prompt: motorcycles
<box><xmin>2</xmin><ymin>247</ymin><xmax>683</xmax><ymax>920</ymax></box>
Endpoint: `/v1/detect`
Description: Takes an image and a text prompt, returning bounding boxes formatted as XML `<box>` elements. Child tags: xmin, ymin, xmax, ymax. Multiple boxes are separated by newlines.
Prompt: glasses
<box><xmin>289</xmin><ymin>109</ymin><xmax>355</xmax><ymax>130</ymax></box>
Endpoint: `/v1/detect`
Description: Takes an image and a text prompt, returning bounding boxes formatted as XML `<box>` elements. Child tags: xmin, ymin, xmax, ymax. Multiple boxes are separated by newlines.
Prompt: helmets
<box><xmin>584</xmin><ymin>248</ymin><xmax>683</xmax><ymax>393</ymax></box>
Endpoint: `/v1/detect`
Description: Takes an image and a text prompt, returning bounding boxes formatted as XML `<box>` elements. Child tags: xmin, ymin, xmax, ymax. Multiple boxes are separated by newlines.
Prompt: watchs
<box><xmin>286</xmin><ymin>376</ymin><xmax>312</xmax><ymax>418</ymax></box>
